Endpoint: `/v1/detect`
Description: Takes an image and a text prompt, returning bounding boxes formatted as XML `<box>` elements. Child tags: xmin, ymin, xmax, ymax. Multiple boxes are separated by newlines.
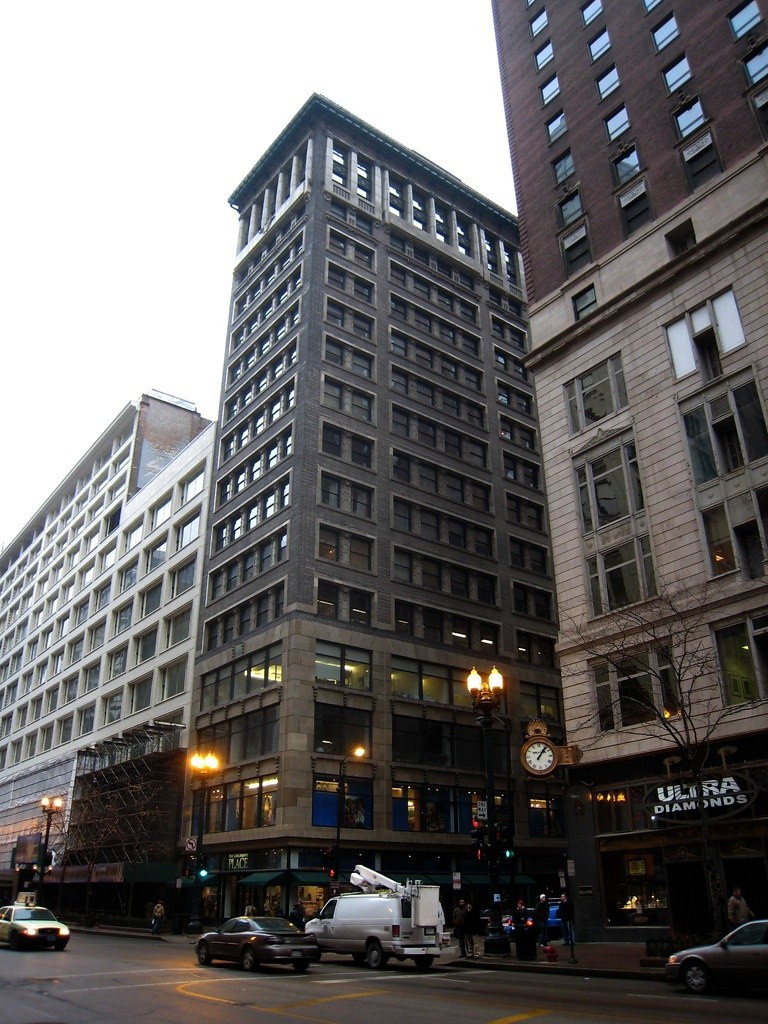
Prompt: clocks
<box><xmin>520</xmin><ymin>737</ymin><xmax>559</xmax><ymax>775</ymax></box>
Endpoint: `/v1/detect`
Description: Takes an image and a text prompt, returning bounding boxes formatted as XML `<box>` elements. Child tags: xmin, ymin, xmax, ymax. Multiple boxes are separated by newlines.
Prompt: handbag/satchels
<box><xmin>151</xmin><ymin>919</ymin><xmax>155</xmax><ymax>924</ymax></box>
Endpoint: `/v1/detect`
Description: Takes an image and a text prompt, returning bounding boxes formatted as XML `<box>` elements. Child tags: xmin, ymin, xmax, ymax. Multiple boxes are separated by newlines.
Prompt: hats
<box><xmin>540</xmin><ymin>894</ymin><xmax>545</xmax><ymax>900</ymax></box>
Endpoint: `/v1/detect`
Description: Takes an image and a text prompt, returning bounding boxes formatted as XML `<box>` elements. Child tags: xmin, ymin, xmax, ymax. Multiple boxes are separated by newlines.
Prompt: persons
<box><xmin>25</xmin><ymin>895</ymin><xmax>37</xmax><ymax>907</ymax></box>
<box><xmin>728</xmin><ymin>887</ymin><xmax>755</xmax><ymax>941</ymax></box>
<box><xmin>151</xmin><ymin>899</ymin><xmax>165</xmax><ymax>934</ymax></box>
<box><xmin>452</xmin><ymin>894</ymin><xmax>550</xmax><ymax>960</ymax></box>
<box><xmin>243</xmin><ymin>896</ymin><xmax>321</xmax><ymax>932</ymax></box>
<box><xmin>426</xmin><ymin>815</ymin><xmax>440</xmax><ymax>831</ymax></box>
<box><xmin>347</xmin><ymin>806</ymin><xmax>364</xmax><ymax>826</ymax></box>
<box><xmin>203</xmin><ymin>895</ymin><xmax>218</xmax><ymax>917</ymax></box>
<box><xmin>558</xmin><ymin>893</ymin><xmax>576</xmax><ymax>946</ymax></box>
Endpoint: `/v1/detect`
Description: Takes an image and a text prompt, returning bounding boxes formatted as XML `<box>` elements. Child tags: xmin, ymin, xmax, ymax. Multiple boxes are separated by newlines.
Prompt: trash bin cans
<box><xmin>513</xmin><ymin>924</ymin><xmax>537</xmax><ymax>960</ymax></box>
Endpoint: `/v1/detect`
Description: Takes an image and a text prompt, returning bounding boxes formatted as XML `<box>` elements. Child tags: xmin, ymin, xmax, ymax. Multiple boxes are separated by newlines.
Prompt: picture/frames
<box><xmin>740</xmin><ymin>676</ymin><xmax>754</xmax><ymax>699</ymax></box>
<box><xmin>728</xmin><ymin>674</ymin><xmax>742</xmax><ymax>698</ymax></box>
<box><xmin>408</xmin><ymin>806</ymin><xmax>415</xmax><ymax>821</ymax></box>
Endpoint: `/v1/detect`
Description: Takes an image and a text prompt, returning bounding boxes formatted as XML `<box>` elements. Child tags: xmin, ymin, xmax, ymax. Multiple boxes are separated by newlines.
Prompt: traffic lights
<box><xmin>200</xmin><ymin>852</ymin><xmax>208</xmax><ymax>878</ymax></box>
<box><xmin>505</xmin><ymin>849</ymin><xmax>511</xmax><ymax>858</ymax></box>
<box><xmin>472</xmin><ymin>821</ymin><xmax>479</xmax><ymax>828</ymax></box>
<box><xmin>328</xmin><ymin>868</ymin><xmax>336</xmax><ymax>878</ymax></box>
<box><xmin>477</xmin><ymin>847</ymin><xmax>481</xmax><ymax>861</ymax></box>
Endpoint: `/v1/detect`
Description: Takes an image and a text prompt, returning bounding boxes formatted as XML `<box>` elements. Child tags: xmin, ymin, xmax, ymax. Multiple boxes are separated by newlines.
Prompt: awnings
<box><xmin>170</xmin><ymin>874</ymin><xmax>220</xmax><ymax>886</ymax></box>
<box><xmin>238</xmin><ymin>871</ymin><xmax>284</xmax><ymax>886</ymax></box>
<box><xmin>291</xmin><ymin>871</ymin><xmax>535</xmax><ymax>884</ymax></box>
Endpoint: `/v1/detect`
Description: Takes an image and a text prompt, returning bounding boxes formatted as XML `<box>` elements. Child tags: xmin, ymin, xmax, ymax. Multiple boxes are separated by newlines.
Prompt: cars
<box><xmin>665</xmin><ymin>919</ymin><xmax>768</xmax><ymax>993</ymax></box>
<box><xmin>0</xmin><ymin>892</ymin><xmax>70</xmax><ymax>951</ymax></box>
<box><xmin>195</xmin><ymin>916</ymin><xmax>322</xmax><ymax>971</ymax></box>
<box><xmin>501</xmin><ymin>905</ymin><xmax>563</xmax><ymax>941</ymax></box>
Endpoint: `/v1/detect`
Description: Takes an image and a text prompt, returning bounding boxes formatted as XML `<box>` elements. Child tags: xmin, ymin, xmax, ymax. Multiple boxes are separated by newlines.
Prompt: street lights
<box><xmin>187</xmin><ymin>750</ymin><xmax>222</xmax><ymax>934</ymax></box>
<box><xmin>334</xmin><ymin>746</ymin><xmax>365</xmax><ymax>881</ymax></box>
<box><xmin>466</xmin><ymin>665</ymin><xmax>512</xmax><ymax>959</ymax></box>
<box><xmin>35</xmin><ymin>794</ymin><xmax>64</xmax><ymax>907</ymax></box>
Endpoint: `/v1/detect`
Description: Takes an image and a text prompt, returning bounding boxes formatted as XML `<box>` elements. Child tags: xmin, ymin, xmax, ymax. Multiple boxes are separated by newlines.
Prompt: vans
<box><xmin>305</xmin><ymin>865</ymin><xmax>450</xmax><ymax>970</ymax></box>
